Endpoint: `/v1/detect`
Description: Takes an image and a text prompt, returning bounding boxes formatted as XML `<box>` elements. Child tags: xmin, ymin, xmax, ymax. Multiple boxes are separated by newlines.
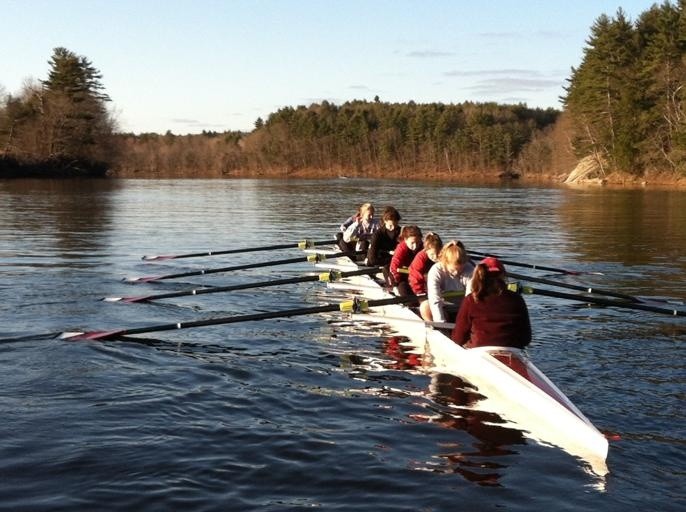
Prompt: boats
<box><xmin>323</xmin><ymin>231</ymin><xmax>609</xmax><ymax>482</ymax></box>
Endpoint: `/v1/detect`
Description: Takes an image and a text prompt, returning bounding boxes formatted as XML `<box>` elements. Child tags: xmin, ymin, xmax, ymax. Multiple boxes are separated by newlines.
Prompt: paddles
<box><xmin>120</xmin><ymin>251</ymin><xmax>369</xmax><ymax>283</ymax></box>
<box><xmin>99</xmin><ymin>265</ymin><xmax>390</xmax><ymax>304</ymax></box>
<box><xmin>506</xmin><ymin>273</ymin><xmax>666</xmax><ymax>306</ymax></box>
<box><xmin>142</xmin><ymin>238</ymin><xmax>339</xmax><ymax>261</ymax></box>
<box><xmin>52</xmin><ymin>292</ymin><xmax>430</xmax><ymax>340</ymax></box>
<box><xmin>469</xmin><ymin>255</ymin><xmax>584</xmax><ymax>275</ymax></box>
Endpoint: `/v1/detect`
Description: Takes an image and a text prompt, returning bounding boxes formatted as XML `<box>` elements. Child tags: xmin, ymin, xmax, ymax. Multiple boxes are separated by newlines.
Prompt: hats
<box><xmin>480</xmin><ymin>257</ymin><xmax>505</xmax><ymax>272</ymax></box>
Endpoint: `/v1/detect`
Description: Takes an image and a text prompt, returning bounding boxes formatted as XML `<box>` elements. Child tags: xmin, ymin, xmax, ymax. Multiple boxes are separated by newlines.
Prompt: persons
<box><xmin>340</xmin><ymin>203</ymin><xmax>475</xmax><ymax>323</ymax></box>
<box><xmin>451</xmin><ymin>256</ymin><xmax>532</xmax><ymax>348</ymax></box>
